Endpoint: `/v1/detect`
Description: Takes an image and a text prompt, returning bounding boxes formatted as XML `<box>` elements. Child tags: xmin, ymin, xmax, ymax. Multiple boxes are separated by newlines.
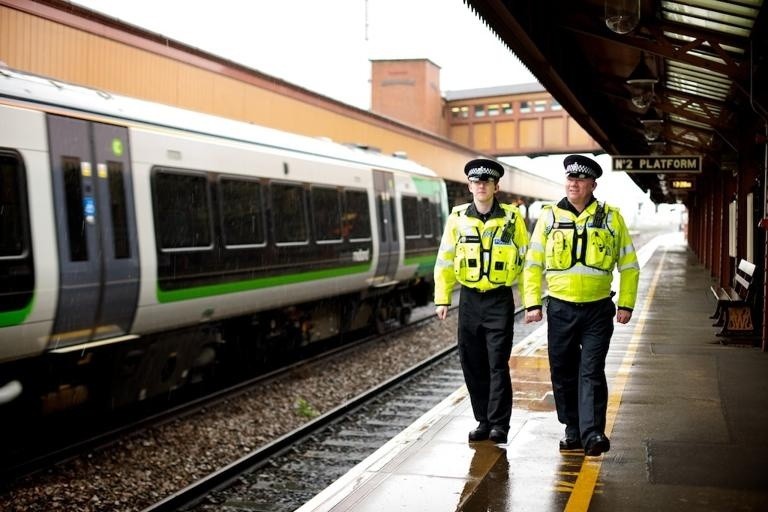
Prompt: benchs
<box><xmin>709</xmin><ymin>257</ymin><xmax>757</xmax><ymax>348</ymax></box>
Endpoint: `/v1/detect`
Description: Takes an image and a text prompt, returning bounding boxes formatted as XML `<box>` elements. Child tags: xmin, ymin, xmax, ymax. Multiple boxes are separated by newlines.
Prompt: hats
<box><xmin>464</xmin><ymin>159</ymin><xmax>504</xmax><ymax>183</ymax></box>
<box><xmin>564</xmin><ymin>155</ymin><xmax>602</xmax><ymax>179</ymax></box>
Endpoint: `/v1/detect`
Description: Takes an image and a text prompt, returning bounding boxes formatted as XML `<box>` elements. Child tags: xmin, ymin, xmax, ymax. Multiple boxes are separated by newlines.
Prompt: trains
<box><xmin>0</xmin><ymin>55</ymin><xmax>451</xmax><ymax>436</ymax></box>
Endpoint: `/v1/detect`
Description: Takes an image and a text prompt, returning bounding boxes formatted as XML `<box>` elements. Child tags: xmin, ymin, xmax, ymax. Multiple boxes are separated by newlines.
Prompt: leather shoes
<box><xmin>560</xmin><ymin>433</ymin><xmax>580</xmax><ymax>449</ymax></box>
<box><xmin>581</xmin><ymin>429</ymin><xmax>610</xmax><ymax>456</ymax></box>
<box><xmin>469</xmin><ymin>421</ymin><xmax>489</xmax><ymax>441</ymax></box>
<box><xmin>489</xmin><ymin>425</ymin><xmax>507</xmax><ymax>443</ymax></box>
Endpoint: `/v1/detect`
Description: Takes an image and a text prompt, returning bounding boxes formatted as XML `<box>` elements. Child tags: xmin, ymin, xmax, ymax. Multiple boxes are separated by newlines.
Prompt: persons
<box><xmin>523</xmin><ymin>155</ymin><xmax>640</xmax><ymax>456</ymax></box>
<box><xmin>434</xmin><ymin>159</ymin><xmax>532</xmax><ymax>445</ymax></box>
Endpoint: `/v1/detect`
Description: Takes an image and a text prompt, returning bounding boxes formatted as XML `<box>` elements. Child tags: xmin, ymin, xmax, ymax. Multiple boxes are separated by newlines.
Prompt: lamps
<box><xmin>588</xmin><ymin>2</ymin><xmax>678</xmax><ymax>197</ymax></box>
